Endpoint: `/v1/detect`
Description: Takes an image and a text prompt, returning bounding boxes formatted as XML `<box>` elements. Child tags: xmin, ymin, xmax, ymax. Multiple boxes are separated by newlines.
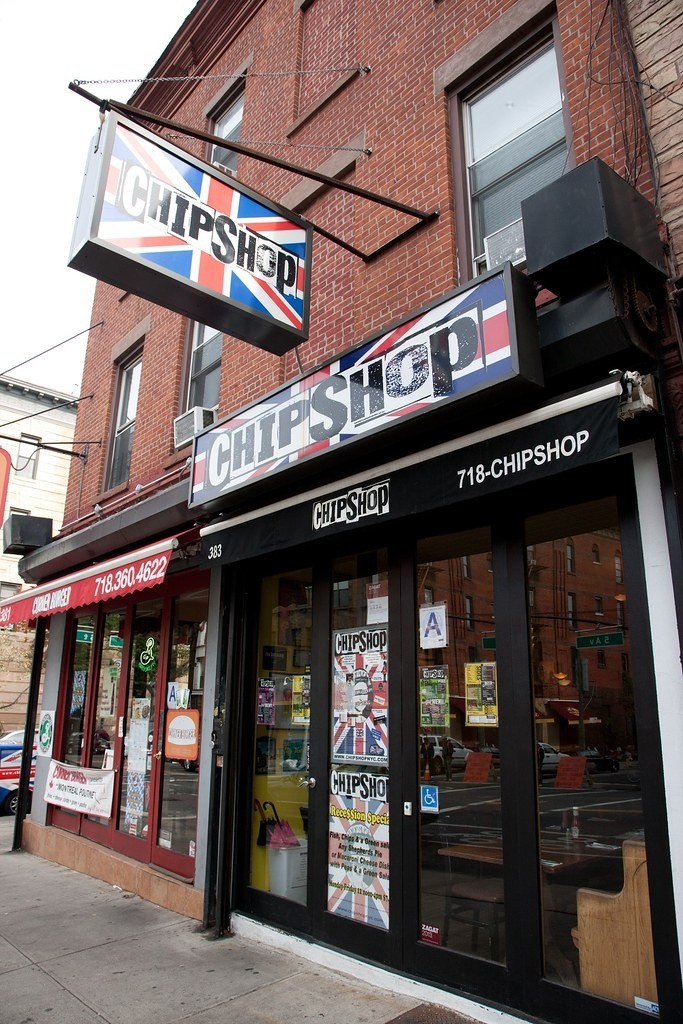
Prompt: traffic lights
<box><xmin>530</xmin><ymin>626</ymin><xmax>539</xmax><ymax>650</ymax></box>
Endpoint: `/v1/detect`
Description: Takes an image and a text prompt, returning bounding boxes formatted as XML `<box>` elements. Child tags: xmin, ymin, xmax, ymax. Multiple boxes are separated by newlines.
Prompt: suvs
<box><xmin>537</xmin><ymin>740</ymin><xmax>573</xmax><ymax>775</ymax></box>
<box><xmin>420</xmin><ymin>735</ymin><xmax>475</xmax><ymax>775</ymax></box>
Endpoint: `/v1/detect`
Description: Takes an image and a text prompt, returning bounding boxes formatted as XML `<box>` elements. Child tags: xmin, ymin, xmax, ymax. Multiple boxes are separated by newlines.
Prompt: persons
<box><xmin>536</xmin><ymin>741</ymin><xmax>546</xmax><ymax>786</ymax></box>
<box><xmin>420</xmin><ymin>736</ymin><xmax>436</xmax><ymax>782</ymax></box>
<box><xmin>442</xmin><ymin>734</ymin><xmax>456</xmax><ymax>780</ymax></box>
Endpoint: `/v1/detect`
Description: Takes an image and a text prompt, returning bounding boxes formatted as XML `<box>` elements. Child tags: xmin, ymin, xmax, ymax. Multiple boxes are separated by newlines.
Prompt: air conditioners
<box><xmin>173</xmin><ymin>406</ymin><xmax>217</xmax><ymax>450</ymax></box>
<box><xmin>483</xmin><ymin>217</ymin><xmax>527</xmax><ymax>270</ymax></box>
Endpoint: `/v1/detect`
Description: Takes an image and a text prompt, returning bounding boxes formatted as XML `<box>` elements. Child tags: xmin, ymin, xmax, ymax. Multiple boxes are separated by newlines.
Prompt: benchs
<box><xmin>570</xmin><ymin>832</ymin><xmax>660</xmax><ymax>1007</ymax></box>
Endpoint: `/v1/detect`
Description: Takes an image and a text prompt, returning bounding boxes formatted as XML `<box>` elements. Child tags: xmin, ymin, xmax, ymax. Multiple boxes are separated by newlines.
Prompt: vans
<box><xmin>66</xmin><ymin>730</ymin><xmax>110</xmax><ymax>754</ymax></box>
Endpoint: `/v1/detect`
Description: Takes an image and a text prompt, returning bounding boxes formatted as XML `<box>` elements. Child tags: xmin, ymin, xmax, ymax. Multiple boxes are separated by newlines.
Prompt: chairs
<box><xmin>437</xmin><ymin>811</ymin><xmax>505</xmax><ymax>962</ymax></box>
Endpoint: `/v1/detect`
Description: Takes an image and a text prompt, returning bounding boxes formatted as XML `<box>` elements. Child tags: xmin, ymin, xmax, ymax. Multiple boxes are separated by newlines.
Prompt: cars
<box><xmin>571</xmin><ymin>750</ymin><xmax>620</xmax><ymax>776</ymax></box>
<box><xmin>472</xmin><ymin>747</ymin><xmax>501</xmax><ymax>768</ymax></box>
<box><xmin>0</xmin><ymin>728</ymin><xmax>40</xmax><ymax>816</ymax></box>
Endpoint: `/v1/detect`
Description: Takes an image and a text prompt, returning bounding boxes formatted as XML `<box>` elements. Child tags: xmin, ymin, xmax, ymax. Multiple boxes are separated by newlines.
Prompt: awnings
<box><xmin>1</xmin><ymin>519</ymin><xmax>208</xmax><ymax>628</ymax></box>
<box><xmin>533</xmin><ymin>700</ymin><xmax>601</xmax><ymax>726</ymax></box>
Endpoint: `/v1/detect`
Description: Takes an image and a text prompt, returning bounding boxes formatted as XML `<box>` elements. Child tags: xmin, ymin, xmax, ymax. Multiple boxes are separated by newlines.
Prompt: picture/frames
<box><xmin>255</xmin><ymin>576</ymin><xmax>312</xmax><ymax>776</ymax></box>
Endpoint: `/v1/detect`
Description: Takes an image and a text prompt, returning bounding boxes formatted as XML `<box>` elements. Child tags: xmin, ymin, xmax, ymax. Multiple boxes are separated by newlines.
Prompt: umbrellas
<box><xmin>262</xmin><ymin>801</ymin><xmax>300</xmax><ymax>846</ymax></box>
<box><xmin>254</xmin><ymin>797</ymin><xmax>278</xmax><ymax>847</ymax></box>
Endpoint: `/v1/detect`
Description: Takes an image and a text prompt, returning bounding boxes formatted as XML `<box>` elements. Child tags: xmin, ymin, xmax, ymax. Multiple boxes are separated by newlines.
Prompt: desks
<box><xmin>438</xmin><ymin>822</ymin><xmax>626</xmax><ymax>952</ymax></box>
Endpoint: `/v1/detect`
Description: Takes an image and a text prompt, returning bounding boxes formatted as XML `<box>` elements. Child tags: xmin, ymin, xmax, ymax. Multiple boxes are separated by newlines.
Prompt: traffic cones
<box><xmin>422</xmin><ymin>762</ymin><xmax>434</xmax><ymax>782</ymax></box>
